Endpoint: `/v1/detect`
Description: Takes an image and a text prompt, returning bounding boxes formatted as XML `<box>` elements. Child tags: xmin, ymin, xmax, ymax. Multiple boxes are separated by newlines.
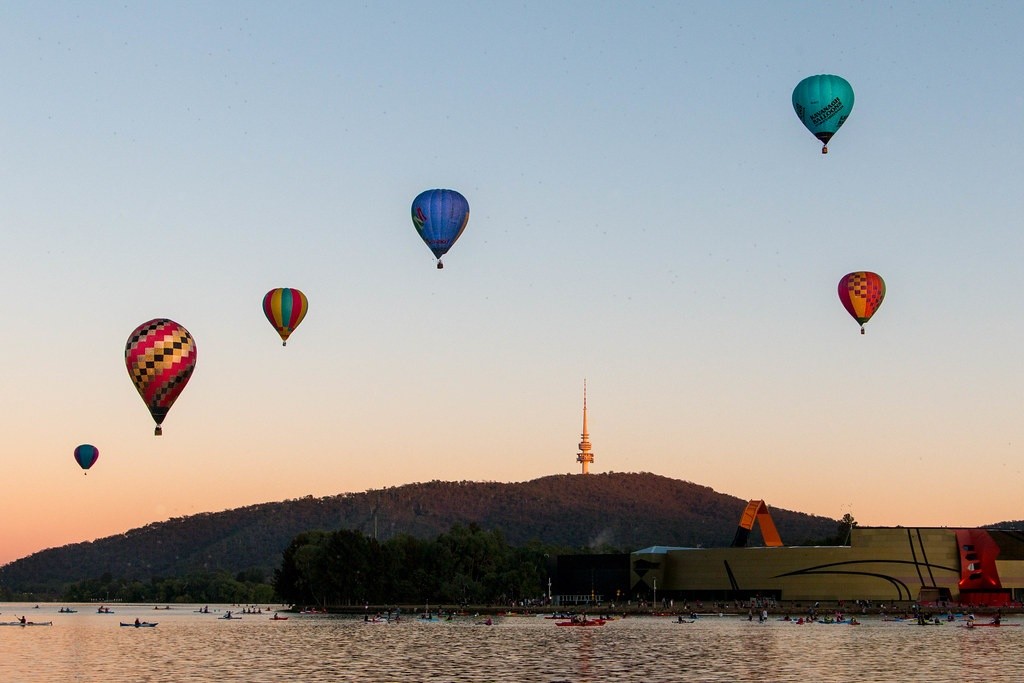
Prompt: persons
<box><xmin>11</xmin><ymin>603</ymin><xmax>1016</xmax><ymax>629</ymax></box>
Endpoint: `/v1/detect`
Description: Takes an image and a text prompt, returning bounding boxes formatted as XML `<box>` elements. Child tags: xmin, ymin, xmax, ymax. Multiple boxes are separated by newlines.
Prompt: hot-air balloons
<box><xmin>125</xmin><ymin>317</ymin><xmax>197</xmax><ymax>438</ymax></box>
<box><xmin>839</xmin><ymin>270</ymin><xmax>886</xmax><ymax>335</ymax></box>
<box><xmin>791</xmin><ymin>74</ymin><xmax>854</xmax><ymax>154</ymax></box>
<box><xmin>74</xmin><ymin>444</ymin><xmax>99</xmax><ymax>476</ymax></box>
<box><xmin>263</xmin><ymin>288</ymin><xmax>308</xmax><ymax>346</ymax></box>
<box><xmin>411</xmin><ymin>189</ymin><xmax>471</xmax><ymax>271</ymax></box>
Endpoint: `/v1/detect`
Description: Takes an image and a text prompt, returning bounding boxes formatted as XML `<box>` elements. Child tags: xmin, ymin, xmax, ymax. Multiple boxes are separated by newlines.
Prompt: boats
<box><xmin>544</xmin><ymin>616</ymin><xmax>572</xmax><ymax>619</ymax></box>
<box><xmin>740</xmin><ymin>613</ymin><xmax>1021</xmax><ymax>627</ymax></box>
<box><xmin>595</xmin><ymin>613</ymin><xmax>703</xmax><ymax>624</ymax></box>
<box><xmin>96</xmin><ymin>611</ymin><xmax>115</xmax><ymax>613</ymax></box>
<box><xmin>237</xmin><ymin>611</ymin><xmax>263</xmax><ymax>614</ymax></box>
<box><xmin>218</xmin><ymin>617</ymin><xmax>243</xmax><ymax>619</ymax></box>
<box><xmin>269</xmin><ymin>617</ymin><xmax>288</xmax><ymax>619</ymax></box>
<box><xmin>119</xmin><ymin>621</ymin><xmax>158</xmax><ymax>627</ymax></box>
<box><xmin>58</xmin><ymin>610</ymin><xmax>77</xmax><ymax>613</ymax></box>
<box><xmin>299</xmin><ymin>611</ymin><xmax>329</xmax><ymax>614</ymax></box>
<box><xmin>0</xmin><ymin>619</ymin><xmax>54</xmax><ymax>626</ymax></box>
<box><xmin>555</xmin><ymin>619</ymin><xmax>606</xmax><ymax>626</ymax></box>
<box><xmin>360</xmin><ymin>613</ymin><xmax>538</xmax><ymax>629</ymax></box>
<box><xmin>153</xmin><ymin>605</ymin><xmax>221</xmax><ymax>615</ymax></box>
